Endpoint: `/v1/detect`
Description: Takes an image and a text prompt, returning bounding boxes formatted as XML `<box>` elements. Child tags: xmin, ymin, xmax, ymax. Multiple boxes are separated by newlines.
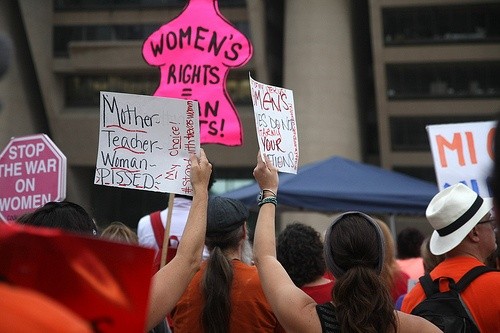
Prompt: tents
<box><xmin>221</xmin><ymin>157</ymin><xmax>441</xmax><ymax>259</ymax></box>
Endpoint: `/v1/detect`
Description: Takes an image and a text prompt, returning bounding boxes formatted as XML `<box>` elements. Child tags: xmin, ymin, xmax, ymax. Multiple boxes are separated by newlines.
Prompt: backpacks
<box><xmin>149</xmin><ymin>211</ymin><xmax>180</xmax><ymax>333</ymax></box>
<box><xmin>410</xmin><ymin>265</ymin><xmax>500</xmax><ymax>333</ymax></box>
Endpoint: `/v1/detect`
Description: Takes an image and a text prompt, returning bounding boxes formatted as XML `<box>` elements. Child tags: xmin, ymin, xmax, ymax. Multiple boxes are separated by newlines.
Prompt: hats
<box><xmin>425</xmin><ymin>183</ymin><xmax>493</xmax><ymax>256</ymax></box>
<box><xmin>206</xmin><ymin>196</ymin><xmax>248</xmax><ymax>233</ymax></box>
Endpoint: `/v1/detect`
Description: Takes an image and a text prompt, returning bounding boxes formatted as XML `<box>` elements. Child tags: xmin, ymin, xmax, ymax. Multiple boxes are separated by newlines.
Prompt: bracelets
<box><xmin>256</xmin><ymin>188</ymin><xmax>277</xmax><ymax>209</ymax></box>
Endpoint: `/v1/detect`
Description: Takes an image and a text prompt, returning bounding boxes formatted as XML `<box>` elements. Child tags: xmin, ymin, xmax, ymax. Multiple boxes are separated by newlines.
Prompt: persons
<box><xmin>373</xmin><ymin>216</ymin><xmax>447</xmax><ymax>315</ymax></box>
<box><xmin>98</xmin><ymin>222</ymin><xmax>140</xmax><ymax>245</ymax></box>
<box><xmin>401</xmin><ymin>183</ymin><xmax>500</xmax><ymax>333</ymax></box>
<box><xmin>276</xmin><ymin>223</ymin><xmax>335</xmax><ymax>305</ymax></box>
<box><xmin>170</xmin><ymin>195</ymin><xmax>287</xmax><ymax>333</ymax></box>
<box><xmin>137</xmin><ymin>160</ymin><xmax>253</xmax><ymax>265</ymax></box>
<box><xmin>13</xmin><ymin>149</ymin><xmax>213</xmax><ymax>333</ymax></box>
<box><xmin>253</xmin><ymin>149</ymin><xmax>444</xmax><ymax>333</ymax></box>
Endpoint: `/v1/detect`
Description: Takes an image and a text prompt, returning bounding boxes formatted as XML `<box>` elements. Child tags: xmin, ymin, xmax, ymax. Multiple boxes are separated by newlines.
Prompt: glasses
<box><xmin>477</xmin><ymin>215</ymin><xmax>497</xmax><ymax>231</ymax></box>
